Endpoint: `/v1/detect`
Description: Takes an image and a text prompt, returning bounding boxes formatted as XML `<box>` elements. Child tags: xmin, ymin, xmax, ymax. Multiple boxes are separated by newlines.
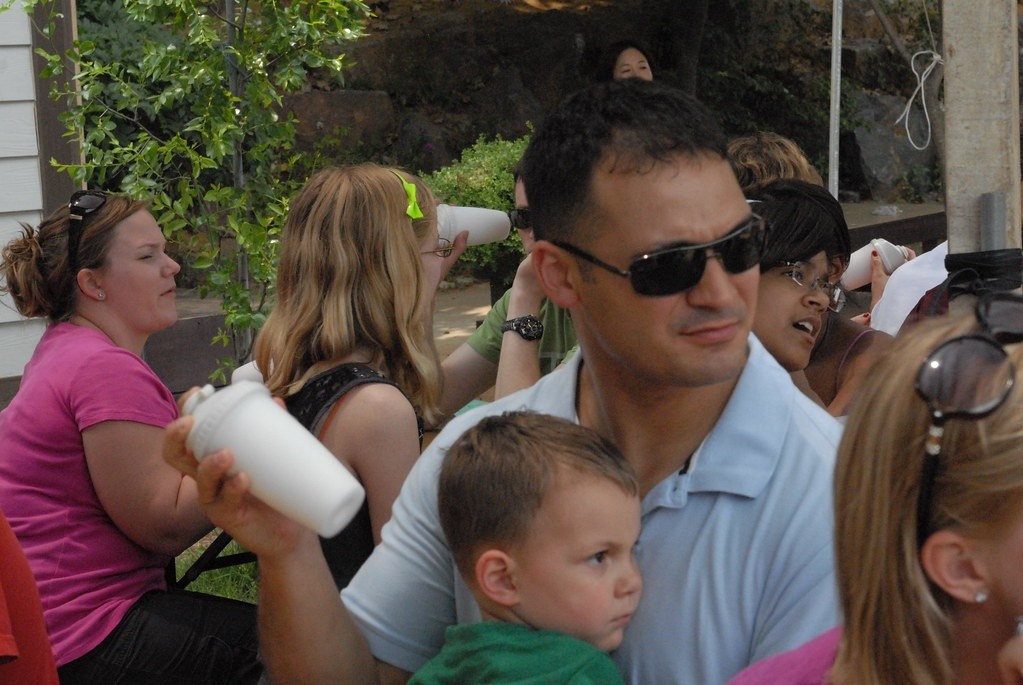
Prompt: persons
<box><xmin>0</xmin><ymin>192</ymin><xmax>262</xmax><ymax>685</ymax></box>
<box><xmin>596</xmin><ymin>41</ymin><xmax>654</xmax><ymax>81</ymax></box>
<box><xmin>424</xmin><ymin>153</ymin><xmax>578</xmax><ymax>430</ymax></box>
<box><xmin>724</xmin><ymin>133</ymin><xmax>947</xmax><ymax>416</ymax></box>
<box><xmin>161</xmin><ymin>81</ymin><xmax>844</xmax><ymax>685</ymax></box>
<box><xmin>244</xmin><ymin>163</ymin><xmax>444</xmax><ymax>589</ymax></box>
<box><xmin>406</xmin><ymin>411</ymin><xmax>641</xmax><ymax>685</ymax></box>
<box><xmin>720</xmin><ymin>301</ymin><xmax>1022</xmax><ymax>685</ymax></box>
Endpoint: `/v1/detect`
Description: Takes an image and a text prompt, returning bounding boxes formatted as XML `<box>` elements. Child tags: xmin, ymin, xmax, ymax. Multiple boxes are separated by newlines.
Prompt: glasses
<box><xmin>760</xmin><ymin>258</ymin><xmax>846</xmax><ymax>313</ymax></box>
<box><xmin>418</xmin><ymin>237</ymin><xmax>452</xmax><ymax>258</ymax></box>
<box><xmin>67</xmin><ymin>189</ymin><xmax>112</xmax><ymax>263</ymax></box>
<box><xmin>551</xmin><ymin>215</ymin><xmax>765</xmax><ymax>297</ymax></box>
<box><xmin>912</xmin><ymin>291</ymin><xmax>1023</xmax><ymax>546</ymax></box>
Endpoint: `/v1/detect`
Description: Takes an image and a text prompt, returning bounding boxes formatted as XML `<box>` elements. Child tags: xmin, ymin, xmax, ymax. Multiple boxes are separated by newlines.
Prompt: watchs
<box><xmin>502</xmin><ymin>314</ymin><xmax>544</xmax><ymax>341</ymax></box>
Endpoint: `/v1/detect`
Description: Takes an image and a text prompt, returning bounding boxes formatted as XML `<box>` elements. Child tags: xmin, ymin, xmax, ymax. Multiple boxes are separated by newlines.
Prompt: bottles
<box><xmin>230</xmin><ymin>358</ymin><xmax>273</xmax><ymax>385</ymax></box>
<box><xmin>840</xmin><ymin>237</ymin><xmax>909</xmax><ymax>291</ymax></box>
<box><xmin>182</xmin><ymin>381</ymin><xmax>365</xmax><ymax>538</ymax></box>
<box><xmin>437</xmin><ymin>203</ymin><xmax>510</xmax><ymax>249</ymax></box>
